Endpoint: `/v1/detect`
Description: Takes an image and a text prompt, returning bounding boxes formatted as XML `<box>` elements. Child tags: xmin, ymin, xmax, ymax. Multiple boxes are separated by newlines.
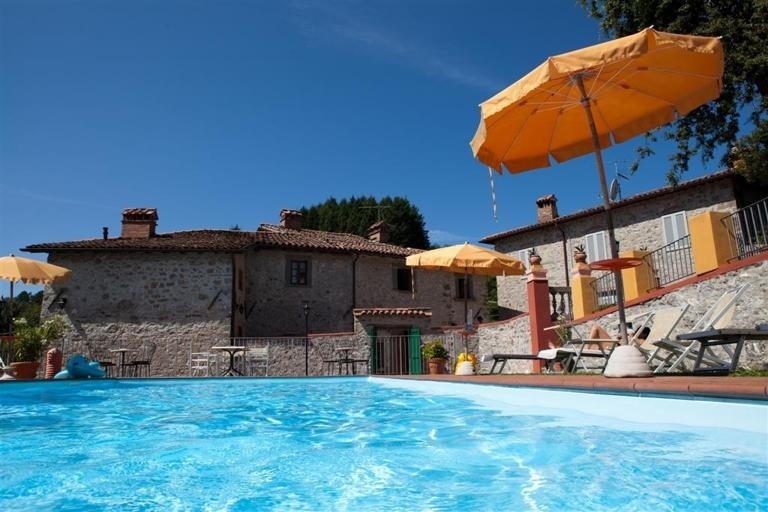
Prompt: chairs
<box><xmin>86</xmin><ymin>342</ymin><xmax>156</xmax><ymax>378</ymax></box>
<box><xmin>318</xmin><ymin>342</ymin><xmax>373</xmax><ymax>375</ymax></box>
<box><xmin>190</xmin><ymin>341</ymin><xmax>270</xmax><ymax>377</ymax></box>
<box><xmin>537</xmin><ymin>283</ymin><xmax>751</xmax><ymax>375</ymax></box>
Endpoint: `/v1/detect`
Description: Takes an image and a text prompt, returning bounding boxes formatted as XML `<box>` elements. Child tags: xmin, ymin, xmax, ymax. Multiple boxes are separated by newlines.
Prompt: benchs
<box><xmin>676</xmin><ymin>328</ymin><xmax>768</xmax><ymax>375</ymax></box>
<box><xmin>483</xmin><ymin>353</ymin><xmax>570</xmax><ymax>375</ymax></box>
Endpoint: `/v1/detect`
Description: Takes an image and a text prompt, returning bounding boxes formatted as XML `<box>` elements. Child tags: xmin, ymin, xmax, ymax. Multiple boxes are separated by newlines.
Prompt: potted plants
<box><xmin>8</xmin><ymin>313</ymin><xmax>70</xmax><ymax>379</ymax></box>
<box><xmin>420</xmin><ymin>338</ymin><xmax>450</xmax><ymax>374</ymax></box>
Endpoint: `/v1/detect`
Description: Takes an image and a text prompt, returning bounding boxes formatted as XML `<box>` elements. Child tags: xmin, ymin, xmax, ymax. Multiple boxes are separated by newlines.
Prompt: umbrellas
<box><xmin>0</xmin><ymin>253</ymin><xmax>72</xmax><ymax>366</ymax></box>
<box><xmin>404</xmin><ymin>240</ymin><xmax>526</xmax><ymax>360</ymax></box>
<box><xmin>467</xmin><ymin>23</ymin><xmax>726</xmax><ymax>347</ymax></box>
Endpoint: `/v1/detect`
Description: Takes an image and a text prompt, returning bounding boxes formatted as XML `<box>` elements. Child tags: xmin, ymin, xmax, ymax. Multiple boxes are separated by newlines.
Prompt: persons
<box><xmin>547</xmin><ymin>325</ymin><xmax>650</xmax><ymax>351</ymax></box>
